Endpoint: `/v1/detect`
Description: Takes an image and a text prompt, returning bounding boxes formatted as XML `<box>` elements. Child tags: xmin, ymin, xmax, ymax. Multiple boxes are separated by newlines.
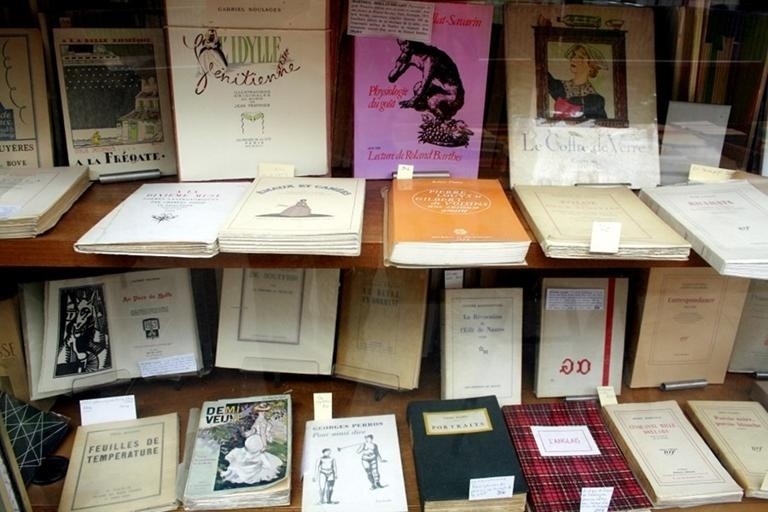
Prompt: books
<box><xmin>0</xmin><ymin>0</ymin><xmax>768</xmax><ymax>512</ymax></box>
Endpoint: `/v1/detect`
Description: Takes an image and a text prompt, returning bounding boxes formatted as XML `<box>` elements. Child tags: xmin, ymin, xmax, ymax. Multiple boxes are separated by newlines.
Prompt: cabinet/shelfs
<box><xmin>0</xmin><ymin>172</ymin><xmax>768</xmax><ymax>512</ymax></box>
